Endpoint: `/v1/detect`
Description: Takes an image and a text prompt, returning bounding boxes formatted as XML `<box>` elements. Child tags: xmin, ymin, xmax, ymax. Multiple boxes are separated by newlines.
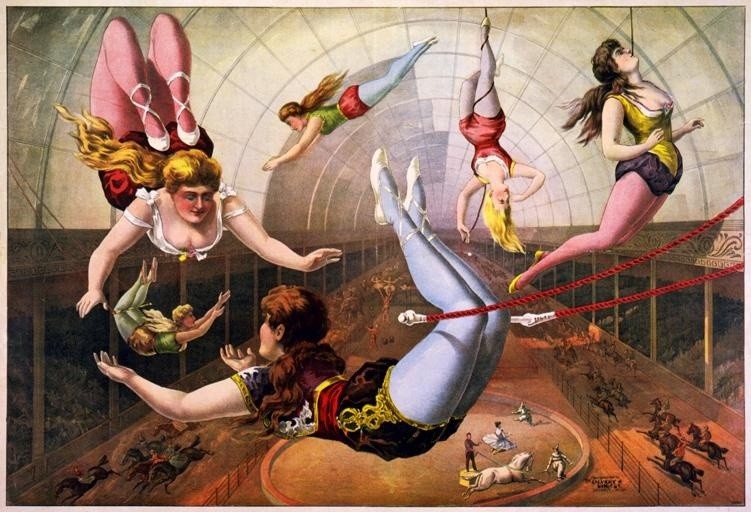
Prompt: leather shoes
<box><xmin>508</xmin><ymin>272</ymin><xmax>524</xmax><ymax>295</ymax></box>
<box><xmin>411</xmin><ymin>34</ymin><xmax>438</xmax><ymax>49</ymax></box>
<box><xmin>528</xmin><ymin>249</ymin><xmax>545</xmax><ymax>269</ymax></box>
<box><xmin>369</xmin><ymin>147</ymin><xmax>438</xmax><ymax>248</ymax></box>
<box><xmin>481</xmin><ymin>16</ymin><xmax>492</xmax><ymax>29</ymax></box>
<box><xmin>140</xmin><ymin>257</ymin><xmax>159</xmax><ymax>286</ymax></box>
<box><xmin>167</xmin><ymin>71</ymin><xmax>201</xmax><ymax>147</ymax></box>
<box><xmin>130</xmin><ymin>83</ymin><xmax>170</xmax><ymax>152</ymax></box>
